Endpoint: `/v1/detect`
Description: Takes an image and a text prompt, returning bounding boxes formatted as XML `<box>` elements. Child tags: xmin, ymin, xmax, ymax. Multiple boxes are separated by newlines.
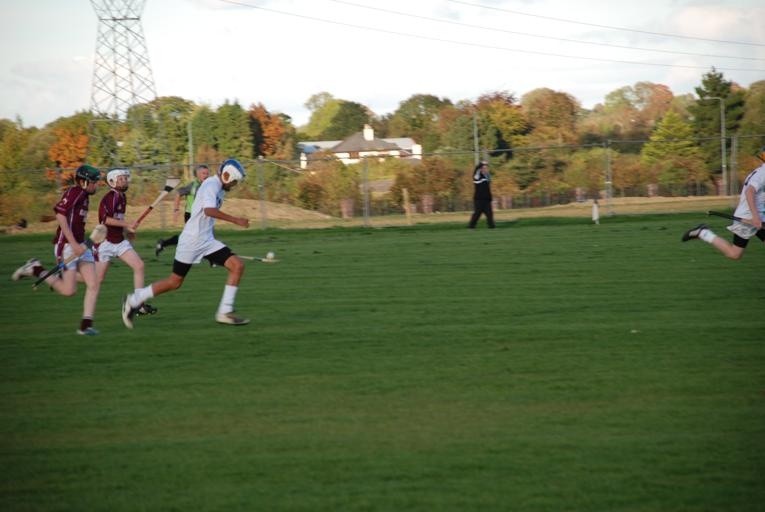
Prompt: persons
<box><xmin>155</xmin><ymin>164</ymin><xmax>215</xmax><ymax>269</ymax></box>
<box><xmin>50</xmin><ymin>169</ymin><xmax>158</xmax><ymax>318</ymax></box>
<box><xmin>11</xmin><ymin>164</ymin><xmax>104</xmax><ymax>337</ymax></box>
<box><xmin>467</xmin><ymin>160</ymin><xmax>496</xmax><ymax>230</ymax></box>
<box><xmin>681</xmin><ymin>148</ymin><xmax>765</xmax><ymax>260</ymax></box>
<box><xmin>121</xmin><ymin>159</ymin><xmax>251</xmax><ymax>330</ymax></box>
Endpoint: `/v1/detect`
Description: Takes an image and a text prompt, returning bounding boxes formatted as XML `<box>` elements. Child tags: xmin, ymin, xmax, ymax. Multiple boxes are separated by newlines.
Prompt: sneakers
<box><xmin>12</xmin><ymin>258</ymin><xmax>42</xmax><ymax>283</ymax></box>
<box><xmin>155</xmin><ymin>239</ymin><xmax>165</xmax><ymax>256</ymax></box>
<box><xmin>76</xmin><ymin>327</ymin><xmax>99</xmax><ymax>335</ymax></box>
<box><xmin>135</xmin><ymin>303</ymin><xmax>160</xmax><ymax>316</ymax></box>
<box><xmin>216</xmin><ymin>311</ymin><xmax>251</xmax><ymax>326</ymax></box>
<box><xmin>682</xmin><ymin>223</ymin><xmax>708</xmax><ymax>241</ymax></box>
<box><xmin>122</xmin><ymin>294</ymin><xmax>136</xmax><ymax>329</ymax></box>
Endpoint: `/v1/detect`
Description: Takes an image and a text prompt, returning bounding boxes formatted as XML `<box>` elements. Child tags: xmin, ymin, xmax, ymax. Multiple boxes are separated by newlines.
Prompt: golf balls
<box><xmin>266</xmin><ymin>252</ymin><xmax>275</xmax><ymax>260</ymax></box>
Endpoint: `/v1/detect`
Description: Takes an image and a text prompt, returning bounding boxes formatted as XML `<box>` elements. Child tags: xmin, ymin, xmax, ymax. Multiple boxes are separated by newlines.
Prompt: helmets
<box><xmin>220</xmin><ymin>160</ymin><xmax>247</xmax><ymax>184</ymax></box>
<box><xmin>107</xmin><ymin>167</ymin><xmax>132</xmax><ymax>187</ymax></box>
<box><xmin>76</xmin><ymin>165</ymin><xmax>104</xmax><ymax>190</ymax></box>
